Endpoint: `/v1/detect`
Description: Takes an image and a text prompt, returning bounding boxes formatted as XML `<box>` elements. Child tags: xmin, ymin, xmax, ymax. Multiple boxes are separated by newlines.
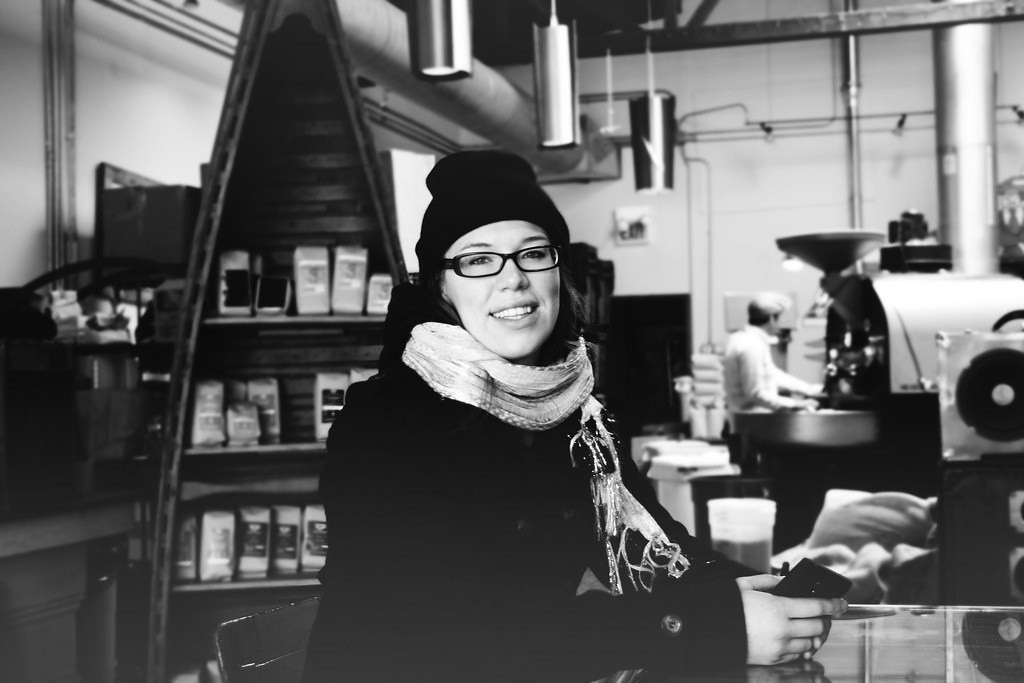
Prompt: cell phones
<box><xmin>772</xmin><ymin>558</ymin><xmax>852</xmax><ymax>599</ymax></box>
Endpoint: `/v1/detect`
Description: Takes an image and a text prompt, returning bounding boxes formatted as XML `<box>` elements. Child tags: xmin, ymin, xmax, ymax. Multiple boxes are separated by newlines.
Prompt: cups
<box><xmin>707</xmin><ymin>498</ymin><xmax>777</xmax><ymax>576</ymax></box>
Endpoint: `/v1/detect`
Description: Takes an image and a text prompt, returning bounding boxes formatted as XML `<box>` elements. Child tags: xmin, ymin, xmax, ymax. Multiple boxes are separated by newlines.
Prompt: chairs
<box><xmin>214</xmin><ymin>587</ymin><xmax>326</xmax><ymax>683</ymax></box>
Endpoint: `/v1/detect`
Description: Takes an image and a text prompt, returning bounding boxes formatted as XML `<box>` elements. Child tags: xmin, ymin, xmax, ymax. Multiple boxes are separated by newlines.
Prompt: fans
<box><xmin>943</xmin><ymin>607</ymin><xmax>1023</xmax><ymax>683</ymax></box>
<box><xmin>935</xmin><ymin>331</ymin><xmax>1024</xmax><ymax>464</ymax></box>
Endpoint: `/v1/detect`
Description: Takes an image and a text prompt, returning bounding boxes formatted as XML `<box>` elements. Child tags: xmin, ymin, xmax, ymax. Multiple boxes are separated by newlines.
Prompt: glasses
<box><xmin>436</xmin><ymin>244</ymin><xmax>563</xmax><ymax>278</ymax></box>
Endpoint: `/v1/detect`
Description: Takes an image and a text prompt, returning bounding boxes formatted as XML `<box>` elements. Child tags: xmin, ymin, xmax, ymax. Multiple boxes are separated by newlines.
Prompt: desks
<box><xmin>605</xmin><ymin>601</ymin><xmax>1024</xmax><ymax>683</ymax></box>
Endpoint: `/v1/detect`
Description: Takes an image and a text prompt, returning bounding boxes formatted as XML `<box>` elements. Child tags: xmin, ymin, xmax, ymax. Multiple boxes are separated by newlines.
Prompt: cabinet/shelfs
<box><xmin>148</xmin><ymin>0</ymin><xmax>413</xmax><ymax>682</ymax></box>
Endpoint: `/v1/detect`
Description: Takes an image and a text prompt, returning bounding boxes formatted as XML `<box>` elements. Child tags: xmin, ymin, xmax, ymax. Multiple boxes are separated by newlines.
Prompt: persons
<box><xmin>721</xmin><ymin>292</ymin><xmax>825</xmax><ymax>552</ymax></box>
<box><xmin>302</xmin><ymin>147</ymin><xmax>848</xmax><ymax>683</ymax></box>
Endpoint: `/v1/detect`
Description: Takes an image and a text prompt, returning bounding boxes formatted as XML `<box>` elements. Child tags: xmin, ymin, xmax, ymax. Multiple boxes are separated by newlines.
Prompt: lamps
<box><xmin>529</xmin><ymin>0</ymin><xmax>583</xmax><ymax>149</ymax></box>
<box><xmin>405</xmin><ymin>0</ymin><xmax>474</xmax><ymax>84</ymax></box>
<box><xmin>627</xmin><ymin>0</ymin><xmax>677</xmax><ymax>195</ymax></box>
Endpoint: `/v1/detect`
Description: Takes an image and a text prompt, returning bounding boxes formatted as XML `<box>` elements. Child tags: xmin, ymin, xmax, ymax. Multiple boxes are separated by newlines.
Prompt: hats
<box><xmin>415</xmin><ymin>150</ymin><xmax>569</xmax><ymax>277</ymax></box>
